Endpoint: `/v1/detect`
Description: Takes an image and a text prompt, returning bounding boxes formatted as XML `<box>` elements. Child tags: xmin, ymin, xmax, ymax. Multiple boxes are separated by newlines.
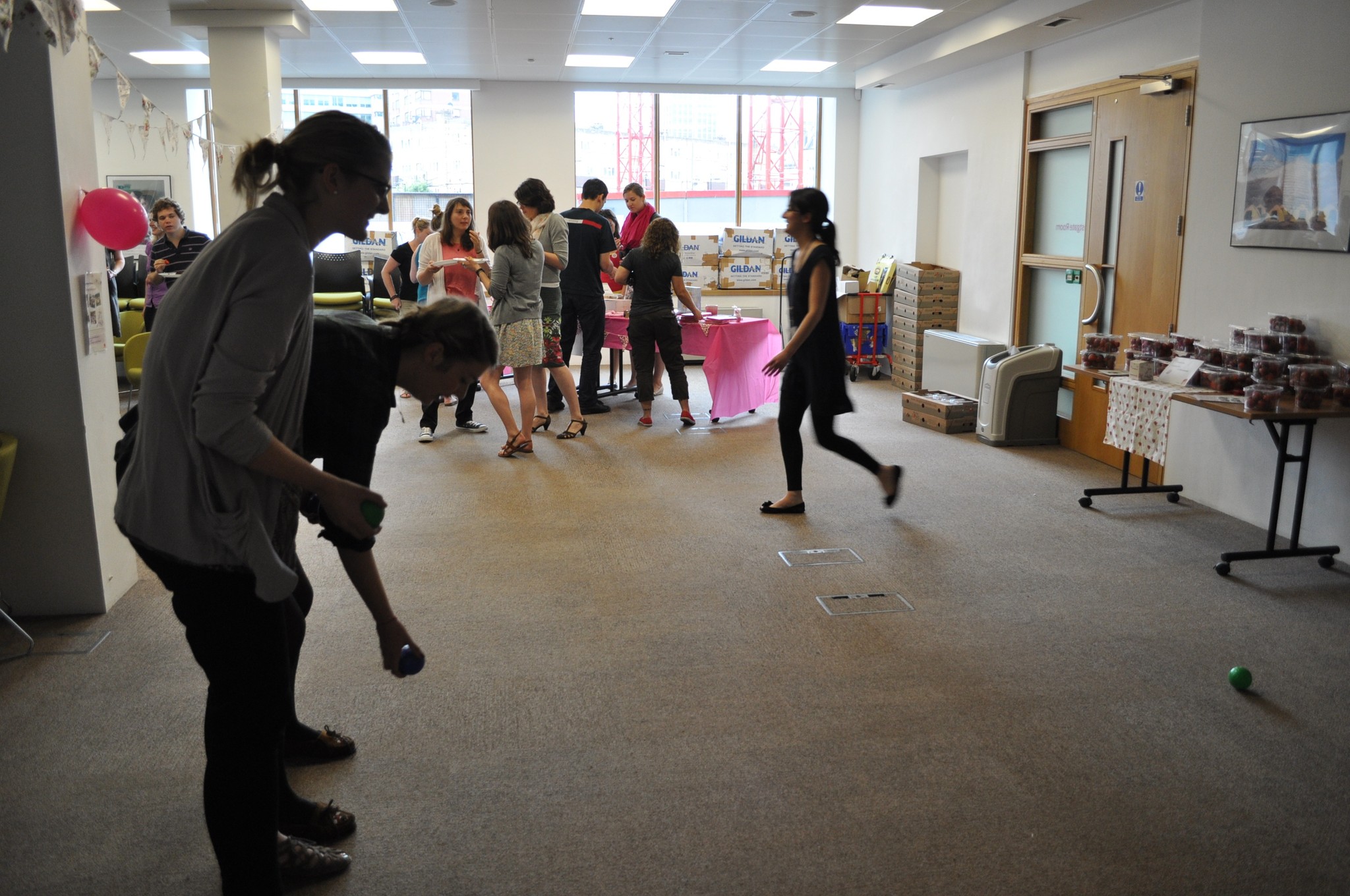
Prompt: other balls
<box><xmin>360</xmin><ymin>498</ymin><xmax>386</xmax><ymax>528</ymax></box>
<box><xmin>398</xmin><ymin>644</ymin><xmax>426</xmax><ymax>676</ymax></box>
<box><xmin>1226</xmin><ymin>667</ymin><xmax>1254</xmax><ymax>691</ymax></box>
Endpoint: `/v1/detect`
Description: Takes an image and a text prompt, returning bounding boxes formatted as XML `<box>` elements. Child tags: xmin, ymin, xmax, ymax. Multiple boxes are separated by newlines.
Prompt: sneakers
<box><xmin>418</xmin><ymin>425</ymin><xmax>433</xmax><ymax>442</ymax></box>
<box><xmin>456</xmin><ymin>419</ymin><xmax>488</xmax><ymax>432</ymax></box>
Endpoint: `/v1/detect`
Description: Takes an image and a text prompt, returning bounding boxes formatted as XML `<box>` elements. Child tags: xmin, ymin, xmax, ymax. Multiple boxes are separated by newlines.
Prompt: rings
<box><xmin>464</xmin><ymin>265</ymin><xmax>467</xmax><ymax>268</ymax></box>
<box><xmin>462</xmin><ymin>262</ymin><xmax>465</xmax><ymax>266</ymax></box>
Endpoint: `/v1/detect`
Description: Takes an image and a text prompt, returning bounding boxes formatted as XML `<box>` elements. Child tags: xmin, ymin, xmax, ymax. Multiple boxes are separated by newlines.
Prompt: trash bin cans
<box><xmin>976</xmin><ymin>342</ymin><xmax>1065</xmax><ymax>448</ymax></box>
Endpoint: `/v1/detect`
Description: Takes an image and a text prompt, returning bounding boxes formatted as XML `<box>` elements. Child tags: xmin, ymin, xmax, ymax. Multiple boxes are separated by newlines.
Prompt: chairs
<box><xmin>111</xmin><ymin>254</ymin><xmax>155</xmax><ymax>413</ymax></box>
<box><xmin>311</xmin><ymin>249</ymin><xmax>401</xmax><ymax>324</ymax></box>
<box><xmin>0</xmin><ymin>432</ymin><xmax>37</xmax><ymax>651</ymax></box>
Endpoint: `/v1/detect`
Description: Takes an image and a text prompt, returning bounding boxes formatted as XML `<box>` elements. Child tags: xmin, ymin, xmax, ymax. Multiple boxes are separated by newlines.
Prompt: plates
<box><xmin>158</xmin><ymin>273</ymin><xmax>182</xmax><ymax>278</ymax></box>
<box><xmin>453</xmin><ymin>257</ymin><xmax>489</xmax><ymax>264</ymax></box>
<box><xmin>431</xmin><ymin>260</ymin><xmax>458</xmax><ymax>266</ymax></box>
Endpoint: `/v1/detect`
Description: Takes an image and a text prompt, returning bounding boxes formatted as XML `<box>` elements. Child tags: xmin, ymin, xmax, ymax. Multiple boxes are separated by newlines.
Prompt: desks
<box><xmin>1061</xmin><ymin>363</ymin><xmax>1350</xmax><ymax>578</ymax></box>
<box><xmin>574</xmin><ymin>311</ymin><xmax>782</xmax><ymax>424</ymax></box>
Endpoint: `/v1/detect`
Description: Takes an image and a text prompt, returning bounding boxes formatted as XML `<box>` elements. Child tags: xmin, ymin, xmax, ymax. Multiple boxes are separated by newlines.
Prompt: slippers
<box><xmin>445</xmin><ymin>397</ymin><xmax>458</xmax><ymax>405</ymax></box>
<box><xmin>399</xmin><ymin>388</ymin><xmax>411</xmax><ymax>398</ymax></box>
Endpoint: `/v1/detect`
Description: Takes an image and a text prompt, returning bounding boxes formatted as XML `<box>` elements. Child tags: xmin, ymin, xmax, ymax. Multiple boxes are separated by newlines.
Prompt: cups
<box><xmin>705</xmin><ymin>305</ymin><xmax>718</xmax><ymax>314</ymax></box>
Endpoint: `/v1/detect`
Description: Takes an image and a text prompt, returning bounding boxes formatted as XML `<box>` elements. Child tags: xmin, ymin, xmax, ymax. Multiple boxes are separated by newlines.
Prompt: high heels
<box><xmin>532</xmin><ymin>414</ymin><xmax>551</xmax><ymax>433</ymax></box>
<box><xmin>557</xmin><ymin>419</ymin><xmax>588</xmax><ymax>439</ymax></box>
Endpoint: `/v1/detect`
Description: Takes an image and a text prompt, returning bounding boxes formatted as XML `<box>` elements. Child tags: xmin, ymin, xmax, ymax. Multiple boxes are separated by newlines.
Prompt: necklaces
<box><xmin>451</xmin><ymin>239</ymin><xmax>461</xmax><ymax>251</ymax></box>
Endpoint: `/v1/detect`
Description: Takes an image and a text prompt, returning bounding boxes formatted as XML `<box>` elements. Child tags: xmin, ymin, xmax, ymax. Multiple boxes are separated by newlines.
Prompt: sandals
<box><xmin>498</xmin><ymin>430</ymin><xmax>529</xmax><ymax>457</ymax></box>
<box><xmin>501</xmin><ymin>440</ymin><xmax>534</xmax><ymax>453</ymax></box>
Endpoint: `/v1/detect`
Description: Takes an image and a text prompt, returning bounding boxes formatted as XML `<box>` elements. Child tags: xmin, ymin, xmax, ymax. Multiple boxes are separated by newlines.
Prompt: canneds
<box><xmin>623</xmin><ymin>310</ymin><xmax>629</xmax><ymax>317</ymax></box>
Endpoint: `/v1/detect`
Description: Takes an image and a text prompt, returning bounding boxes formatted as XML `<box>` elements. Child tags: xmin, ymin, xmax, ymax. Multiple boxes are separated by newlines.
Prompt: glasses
<box><xmin>321</xmin><ymin>166</ymin><xmax>391</xmax><ymax>197</ymax></box>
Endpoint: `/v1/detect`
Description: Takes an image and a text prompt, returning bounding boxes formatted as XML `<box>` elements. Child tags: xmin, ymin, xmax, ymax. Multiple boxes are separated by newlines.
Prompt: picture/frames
<box><xmin>106</xmin><ymin>175</ymin><xmax>172</xmax><ymax>250</ymax></box>
<box><xmin>1227</xmin><ymin>110</ymin><xmax>1350</xmax><ymax>254</ymax></box>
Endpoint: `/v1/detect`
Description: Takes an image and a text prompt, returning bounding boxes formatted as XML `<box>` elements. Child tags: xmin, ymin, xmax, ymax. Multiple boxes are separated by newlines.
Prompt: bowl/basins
<box><xmin>1080</xmin><ymin>311</ymin><xmax>1349</xmax><ymax>413</ymax></box>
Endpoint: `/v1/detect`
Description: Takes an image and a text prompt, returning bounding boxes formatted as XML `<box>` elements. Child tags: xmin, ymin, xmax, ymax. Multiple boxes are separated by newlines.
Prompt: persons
<box><xmin>598</xmin><ymin>209</ymin><xmax>626</xmax><ymax>388</ymax></box>
<box><xmin>514</xmin><ymin>177</ymin><xmax>587</xmax><ymax>439</ymax></box>
<box><xmin>104</xmin><ymin>197</ymin><xmax>212</xmax><ymax>337</ymax></box>
<box><xmin>619</xmin><ymin>183</ymin><xmax>665</xmax><ymax>395</ymax></box>
<box><xmin>114</xmin><ymin>110</ymin><xmax>391</xmax><ymax>896</ymax></box>
<box><xmin>458</xmin><ymin>200</ymin><xmax>545</xmax><ymax>457</ymax></box>
<box><xmin>615</xmin><ymin>217</ymin><xmax>704</xmax><ymax>426</ymax></box>
<box><xmin>759</xmin><ymin>188</ymin><xmax>903</xmax><ymax>513</ymax></box>
<box><xmin>381</xmin><ymin>217</ymin><xmax>434</xmax><ymax>398</ymax></box>
<box><xmin>546</xmin><ymin>179</ymin><xmax>619</xmax><ymax>415</ymax></box>
<box><xmin>116</xmin><ymin>294</ymin><xmax>501</xmax><ymax>847</ymax></box>
<box><xmin>131</xmin><ymin>190</ymin><xmax>156</xmax><ymax>238</ymax></box>
<box><xmin>410</xmin><ymin>197</ymin><xmax>492</xmax><ymax>442</ymax></box>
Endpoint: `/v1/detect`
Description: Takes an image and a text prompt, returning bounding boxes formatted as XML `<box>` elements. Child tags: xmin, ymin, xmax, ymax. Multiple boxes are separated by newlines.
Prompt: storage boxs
<box><xmin>717</xmin><ymin>256</ymin><xmax>772</xmax><ymax>290</ymax></box>
<box><xmin>772</xmin><ymin>227</ymin><xmax>801</xmax><ymax>259</ymax></box>
<box><xmin>361</xmin><ymin>260</ymin><xmax>375</xmax><ymax>292</ymax></box>
<box><xmin>670</xmin><ymin>234</ymin><xmax>719</xmax><ymax>266</ymax></box>
<box><xmin>671</xmin><ymin>265</ymin><xmax>718</xmax><ymax>290</ymax></box>
<box><xmin>718</xmin><ymin>226</ymin><xmax>774</xmax><ymax>257</ymax></box>
<box><xmin>835</xmin><ymin>260</ymin><xmax>978</xmax><ymax>435</ymax></box>
<box><xmin>344</xmin><ymin>230</ymin><xmax>398</xmax><ymax>261</ymax></box>
<box><xmin>771</xmin><ymin>258</ymin><xmax>793</xmax><ymax>290</ymax></box>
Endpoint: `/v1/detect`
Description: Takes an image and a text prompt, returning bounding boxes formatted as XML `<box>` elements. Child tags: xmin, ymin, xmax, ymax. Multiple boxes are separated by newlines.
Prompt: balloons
<box><xmin>80</xmin><ymin>186</ymin><xmax>149</xmax><ymax>251</ymax></box>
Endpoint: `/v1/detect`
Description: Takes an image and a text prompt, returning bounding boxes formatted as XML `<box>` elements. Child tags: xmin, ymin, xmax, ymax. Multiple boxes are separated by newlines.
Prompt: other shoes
<box><xmin>639</xmin><ymin>416</ymin><xmax>652</xmax><ymax>426</ymax></box>
<box><xmin>634</xmin><ymin>384</ymin><xmax>664</xmax><ymax>398</ymax></box>
<box><xmin>547</xmin><ymin>400</ymin><xmax>565</xmax><ymax>411</ymax></box>
<box><xmin>581</xmin><ymin>401</ymin><xmax>610</xmax><ymax>415</ymax></box>
<box><xmin>760</xmin><ymin>501</ymin><xmax>805</xmax><ymax>513</ymax></box>
<box><xmin>285</xmin><ymin>728</ymin><xmax>356</xmax><ymax>760</ymax></box>
<box><xmin>270</xmin><ymin>798</ymin><xmax>355</xmax><ymax>836</ymax></box>
<box><xmin>680</xmin><ymin>410</ymin><xmax>696</xmax><ymax>424</ymax></box>
<box><xmin>887</xmin><ymin>464</ymin><xmax>901</xmax><ymax>505</ymax></box>
<box><xmin>623</xmin><ymin>385</ymin><xmax>637</xmax><ymax>389</ymax></box>
<box><xmin>223</xmin><ymin>831</ymin><xmax>351</xmax><ymax>896</ymax></box>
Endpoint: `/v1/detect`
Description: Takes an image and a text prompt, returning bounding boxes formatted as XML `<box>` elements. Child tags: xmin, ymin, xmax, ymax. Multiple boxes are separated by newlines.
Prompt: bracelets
<box><xmin>390</xmin><ymin>294</ymin><xmax>398</xmax><ymax>301</ymax></box>
<box><xmin>476</xmin><ymin>250</ymin><xmax>482</xmax><ymax>254</ymax></box>
<box><xmin>476</xmin><ymin>268</ymin><xmax>485</xmax><ymax>276</ymax></box>
